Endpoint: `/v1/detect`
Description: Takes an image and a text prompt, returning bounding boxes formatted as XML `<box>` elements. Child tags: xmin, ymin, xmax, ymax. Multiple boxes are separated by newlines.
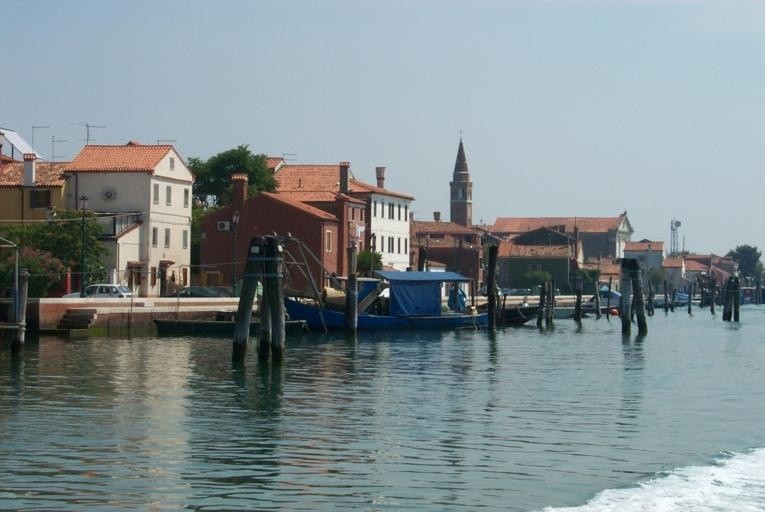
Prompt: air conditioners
<box><xmin>103</xmin><ymin>190</ymin><xmax>116</xmax><ymax>201</ymax></box>
<box><xmin>217</xmin><ymin>222</ymin><xmax>231</xmax><ymax>231</ymax></box>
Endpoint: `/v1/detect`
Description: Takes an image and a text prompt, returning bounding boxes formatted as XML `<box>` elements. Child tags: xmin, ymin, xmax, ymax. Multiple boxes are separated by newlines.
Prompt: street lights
<box><xmin>232</xmin><ymin>210</ymin><xmax>240</xmax><ymax>297</ymax></box>
<box><xmin>79</xmin><ymin>191</ymin><xmax>89</xmax><ymax>298</ymax></box>
<box><xmin>425</xmin><ymin>231</ymin><xmax>430</xmax><ymax>272</ymax></box>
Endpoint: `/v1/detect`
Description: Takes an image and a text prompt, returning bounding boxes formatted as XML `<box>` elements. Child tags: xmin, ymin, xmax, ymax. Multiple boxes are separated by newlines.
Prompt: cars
<box><xmin>166</xmin><ymin>286</ymin><xmax>237</xmax><ymax>298</ymax></box>
<box><xmin>63</xmin><ymin>283</ymin><xmax>132</xmax><ymax>298</ymax></box>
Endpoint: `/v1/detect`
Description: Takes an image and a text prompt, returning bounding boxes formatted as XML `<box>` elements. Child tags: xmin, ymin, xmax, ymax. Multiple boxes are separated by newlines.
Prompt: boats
<box><xmin>154</xmin><ymin>319</ymin><xmax>309</xmax><ymax>339</ymax></box>
<box><xmin>579</xmin><ymin>287</ymin><xmax>621</xmax><ymax>310</ymax></box>
<box><xmin>478</xmin><ymin>306</ymin><xmax>540</xmax><ymax>326</ymax></box>
<box><xmin>283</xmin><ymin>269</ymin><xmax>490</xmax><ymax>330</ymax></box>
<box><xmin>541</xmin><ymin>307</ymin><xmax>592</xmax><ymax>318</ymax></box>
